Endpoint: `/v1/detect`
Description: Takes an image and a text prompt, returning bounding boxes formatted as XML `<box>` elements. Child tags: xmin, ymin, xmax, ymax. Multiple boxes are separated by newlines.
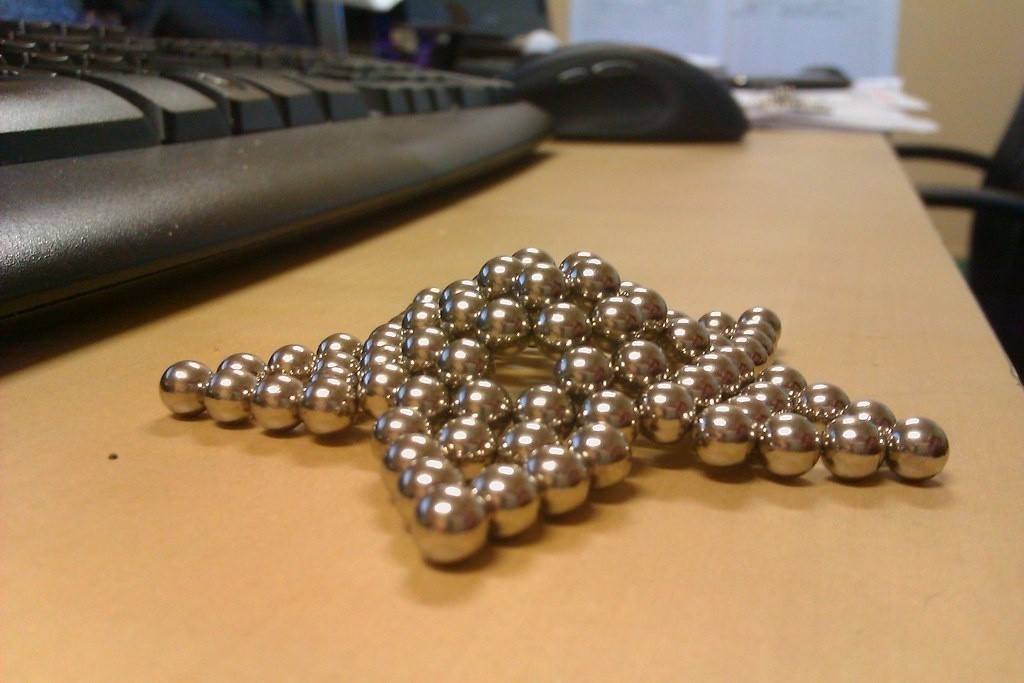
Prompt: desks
<box><xmin>0</xmin><ymin>127</ymin><xmax>1024</xmax><ymax>683</ymax></box>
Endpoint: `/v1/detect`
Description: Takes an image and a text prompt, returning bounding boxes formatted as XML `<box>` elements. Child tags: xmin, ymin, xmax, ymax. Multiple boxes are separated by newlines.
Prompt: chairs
<box><xmin>894</xmin><ymin>90</ymin><xmax>1024</xmax><ymax>386</ymax></box>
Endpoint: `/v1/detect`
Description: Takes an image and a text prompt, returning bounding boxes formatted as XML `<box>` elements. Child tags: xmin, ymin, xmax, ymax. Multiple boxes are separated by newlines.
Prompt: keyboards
<box><xmin>0</xmin><ymin>19</ymin><xmax>553</xmax><ymax>337</ymax></box>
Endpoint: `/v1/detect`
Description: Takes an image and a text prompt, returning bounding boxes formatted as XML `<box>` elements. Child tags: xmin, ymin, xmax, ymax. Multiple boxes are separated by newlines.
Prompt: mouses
<box><xmin>493</xmin><ymin>44</ymin><xmax>748</xmax><ymax>144</ymax></box>
